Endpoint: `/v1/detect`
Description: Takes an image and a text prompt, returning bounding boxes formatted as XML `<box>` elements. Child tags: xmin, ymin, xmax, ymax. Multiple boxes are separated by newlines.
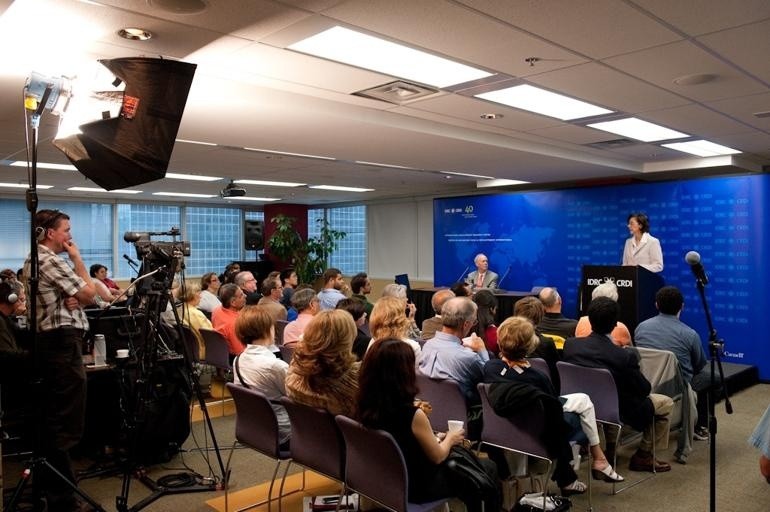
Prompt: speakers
<box><xmin>245</xmin><ymin>220</ymin><xmax>264</xmax><ymax>250</ymax></box>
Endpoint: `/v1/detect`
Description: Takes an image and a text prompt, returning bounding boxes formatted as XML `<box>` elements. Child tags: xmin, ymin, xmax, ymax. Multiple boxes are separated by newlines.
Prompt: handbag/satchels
<box><xmin>510</xmin><ymin>491</ymin><xmax>571</xmax><ymax>512</ymax></box>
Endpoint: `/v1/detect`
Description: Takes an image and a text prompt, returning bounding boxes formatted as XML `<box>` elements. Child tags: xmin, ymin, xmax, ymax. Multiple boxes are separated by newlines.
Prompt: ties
<box><xmin>476</xmin><ymin>275</ymin><xmax>483</xmax><ymax>288</ymax></box>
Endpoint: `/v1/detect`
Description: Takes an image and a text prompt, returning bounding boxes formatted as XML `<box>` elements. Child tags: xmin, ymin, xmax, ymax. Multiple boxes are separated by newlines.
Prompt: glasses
<box><xmin>209</xmin><ymin>278</ymin><xmax>219</xmax><ymax>282</ymax></box>
<box><xmin>470</xmin><ymin>318</ymin><xmax>478</xmax><ymax>326</ymax></box>
<box><xmin>233</xmin><ymin>266</ymin><xmax>241</xmax><ymax>270</ymax></box>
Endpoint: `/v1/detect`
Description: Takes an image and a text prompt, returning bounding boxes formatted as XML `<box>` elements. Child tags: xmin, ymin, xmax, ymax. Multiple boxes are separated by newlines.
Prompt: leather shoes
<box><xmin>627</xmin><ymin>454</ymin><xmax>671</xmax><ymax>473</ymax></box>
<box><xmin>67</xmin><ymin>459</ymin><xmax>108</xmax><ymax>478</ymax></box>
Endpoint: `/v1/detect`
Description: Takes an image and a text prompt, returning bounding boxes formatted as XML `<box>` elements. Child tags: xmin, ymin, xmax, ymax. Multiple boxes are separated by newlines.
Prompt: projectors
<box><xmin>223</xmin><ymin>188</ymin><xmax>246</xmax><ymax>197</ymax></box>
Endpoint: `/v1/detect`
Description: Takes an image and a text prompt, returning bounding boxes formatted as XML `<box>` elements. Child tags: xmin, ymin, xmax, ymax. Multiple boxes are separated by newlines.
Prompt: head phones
<box><xmin>35</xmin><ymin>209</ymin><xmax>60</xmax><ymax>241</ymax></box>
<box><xmin>0</xmin><ymin>277</ymin><xmax>17</xmax><ymax>304</ymax></box>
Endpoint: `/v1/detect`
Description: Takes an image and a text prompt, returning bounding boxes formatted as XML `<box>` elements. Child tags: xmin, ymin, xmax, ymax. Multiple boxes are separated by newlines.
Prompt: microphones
<box><xmin>455</xmin><ymin>266</ymin><xmax>470</xmax><ymax>282</ymax></box>
<box><xmin>493</xmin><ymin>264</ymin><xmax>512</xmax><ymax>294</ymax></box>
<box><xmin>123</xmin><ymin>254</ymin><xmax>139</xmax><ymax>266</ymax></box>
<box><xmin>686</xmin><ymin>251</ymin><xmax>709</xmax><ymax>285</ymax></box>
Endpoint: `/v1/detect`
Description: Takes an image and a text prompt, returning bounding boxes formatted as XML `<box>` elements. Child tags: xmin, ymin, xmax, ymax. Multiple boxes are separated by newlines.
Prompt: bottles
<box><xmin>93</xmin><ymin>334</ymin><xmax>107</xmax><ymax>365</ymax></box>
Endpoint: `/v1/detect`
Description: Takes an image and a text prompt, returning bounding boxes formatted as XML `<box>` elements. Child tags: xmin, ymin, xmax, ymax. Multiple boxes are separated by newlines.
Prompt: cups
<box><xmin>116</xmin><ymin>349</ymin><xmax>129</xmax><ymax>357</ymax></box>
<box><xmin>447</xmin><ymin>420</ymin><xmax>465</xmax><ymax>434</ymax></box>
<box><xmin>462</xmin><ymin>336</ymin><xmax>473</xmax><ymax>345</ymax></box>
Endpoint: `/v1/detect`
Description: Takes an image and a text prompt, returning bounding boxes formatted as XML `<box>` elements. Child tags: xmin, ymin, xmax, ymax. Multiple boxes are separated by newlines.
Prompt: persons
<box><xmin>23</xmin><ymin>208</ymin><xmax>103</xmax><ymax>511</ymax></box>
<box><xmin>620</xmin><ymin>212</ymin><xmax>665</xmax><ymax>275</ymax></box>
<box><xmin>749</xmin><ymin>404</ymin><xmax>770</xmax><ymax>484</ymax></box>
<box><xmin>462</xmin><ymin>252</ymin><xmax>498</xmax><ymax>292</ymax></box>
<box><xmin>0</xmin><ymin>263</ymin><xmax>705</xmax><ymax>512</ymax></box>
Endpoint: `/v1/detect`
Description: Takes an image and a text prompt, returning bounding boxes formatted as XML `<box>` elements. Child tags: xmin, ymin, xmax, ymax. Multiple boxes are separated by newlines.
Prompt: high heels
<box><xmin>558</xmin><ymin>479</ymin><xmax>588</xmax><ymax>497</ymax></box>
<box><xmin>590</xmin><ymin>463</ymin><xmax>625</xmax><ymax>483</ymax></box>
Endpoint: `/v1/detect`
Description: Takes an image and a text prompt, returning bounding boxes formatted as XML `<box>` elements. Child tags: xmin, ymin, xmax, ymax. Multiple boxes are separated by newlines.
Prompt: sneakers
<box><xmin>692</xmin><ymin>424</ymin><xmax>710</xmax><ymax>441</ymax></box>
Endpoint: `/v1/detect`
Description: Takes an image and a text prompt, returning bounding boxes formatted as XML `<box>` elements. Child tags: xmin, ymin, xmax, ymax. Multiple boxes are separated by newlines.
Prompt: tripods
<box><xmin>0</xmin><ymin>112</ymin><xmax>105</xmax><ymax>512</ymax></box>
<box><xmin>113</xmin><ymin>290</ymin><xmax>229</xmax><ymax>512</ymax></box>
